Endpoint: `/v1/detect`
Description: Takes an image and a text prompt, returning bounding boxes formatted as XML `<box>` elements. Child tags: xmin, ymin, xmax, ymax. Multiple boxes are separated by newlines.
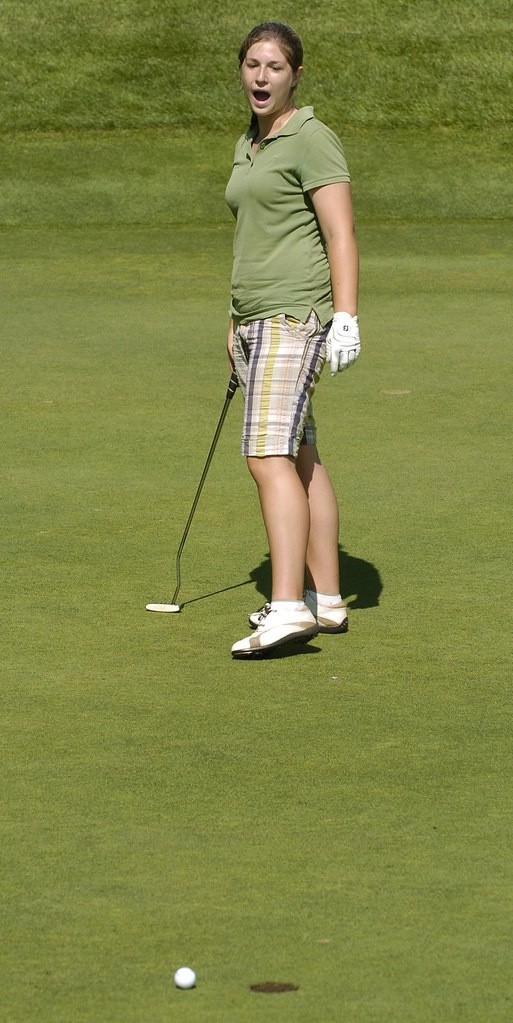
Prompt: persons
<box><xmin>223</xmin><ymin>22</ymin><xmax>362</xmax><ymax>657</ymax></box>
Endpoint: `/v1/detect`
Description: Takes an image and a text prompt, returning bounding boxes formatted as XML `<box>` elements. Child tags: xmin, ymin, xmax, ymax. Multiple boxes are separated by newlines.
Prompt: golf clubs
<box><xmin>145</xmin><ymin>365</ymin><xmax>238</xmax><ymax>613</ymax></box>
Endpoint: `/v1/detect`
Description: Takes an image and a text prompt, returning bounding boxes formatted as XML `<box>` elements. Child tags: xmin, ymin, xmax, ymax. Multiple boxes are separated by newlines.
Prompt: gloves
<box><xmin>325</xmin><ymin>312</ymin><xmax>361</xmax><ymax>377</ymax></box>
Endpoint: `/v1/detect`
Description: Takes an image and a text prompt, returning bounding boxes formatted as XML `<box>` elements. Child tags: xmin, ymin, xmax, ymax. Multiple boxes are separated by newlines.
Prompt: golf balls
<box><xmin>174</xmin><ymin>967</ymin><xmax>197</xmax><ymax>989</ymax></box>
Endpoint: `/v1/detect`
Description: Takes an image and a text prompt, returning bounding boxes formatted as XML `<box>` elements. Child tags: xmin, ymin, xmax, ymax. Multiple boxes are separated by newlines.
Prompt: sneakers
<box><xmin>232</xmin><ymin>603</ymin><xmax>320</xmax><ymax>658</ymax></box>
<box><xmin>249</xmin><ymin>590</ymin><xmax>348</xmax><ymax>634</ymax></box>
